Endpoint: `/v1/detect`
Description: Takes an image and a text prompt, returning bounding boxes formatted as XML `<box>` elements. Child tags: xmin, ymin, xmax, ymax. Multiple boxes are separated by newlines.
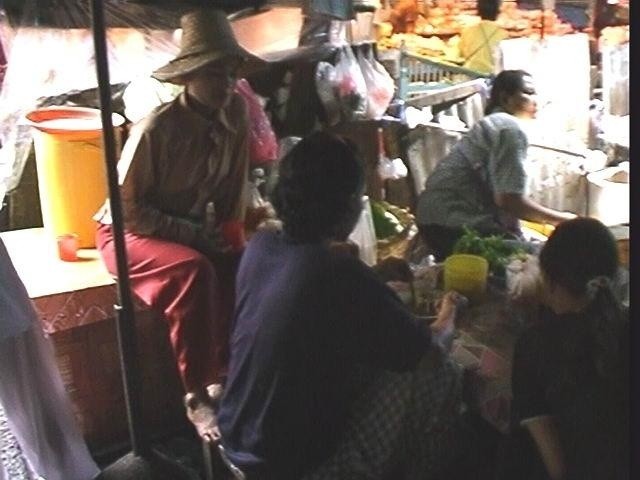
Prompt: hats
<box><xmin>150</xmin><ymin>11</ymin><xmax>270</xmax><ymax>84</ymax></box>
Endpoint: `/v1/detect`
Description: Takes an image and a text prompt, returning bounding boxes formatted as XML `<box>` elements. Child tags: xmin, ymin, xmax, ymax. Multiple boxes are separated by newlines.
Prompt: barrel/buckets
<box><xmin>24</xmin><ymin>106</ymin><xmax>128</xmax><ymax>249</ymax></box>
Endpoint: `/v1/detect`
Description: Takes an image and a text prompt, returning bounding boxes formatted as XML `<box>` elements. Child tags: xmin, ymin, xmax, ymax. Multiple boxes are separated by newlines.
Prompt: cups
<box><xmin>444</xmin><ymin>255</ymin><xmax>488</xmax><ymax>305</ymax></box>
<box><xmin>407</xmin><ymin>269</ymin><xmax>436</xmax><ymax>316</ymax></box>
<box><xmin>222</xmin><ymin>222</ymin><xmax>243</xmax><ymax>248</ymax></box>
<box><xmin>57</xmin><ymin>234</ymin><xmax>78</xmax><ymax>262</ymax></box>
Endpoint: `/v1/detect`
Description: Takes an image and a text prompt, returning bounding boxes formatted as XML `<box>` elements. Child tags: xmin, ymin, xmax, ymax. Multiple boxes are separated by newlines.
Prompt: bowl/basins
<box><xmin>24</xmin><ymin>106</ymin><xmax>126</xmax><ymax>133</ymax></box>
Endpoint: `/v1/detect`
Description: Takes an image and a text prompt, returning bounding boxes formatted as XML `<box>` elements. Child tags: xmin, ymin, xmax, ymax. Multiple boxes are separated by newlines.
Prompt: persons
<box><xmin>94</xmin><ymin>11</ymin><xmax>274</xmax><ymax>444</ymax></box>
<box><xmin>496</xmin><ymin>219</ymin><xmax>631</xmax><ymax>478</ymax></box>
<box><xmin>219</xmin><ymin>130</ymin><xmax>468</xmax><ymax>478</ymax></box>
<box><xmin>417</xmin><ymin>70</ymin><xmax>578</xmax><ymax>264</ymax></box>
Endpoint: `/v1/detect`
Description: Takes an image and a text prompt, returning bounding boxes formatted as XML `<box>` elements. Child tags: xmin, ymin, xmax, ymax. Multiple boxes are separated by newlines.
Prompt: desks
<box><xmin>423</xmin><ymin>292</ymin><xmax>540</xmax><ymax>441</ymax></box>
